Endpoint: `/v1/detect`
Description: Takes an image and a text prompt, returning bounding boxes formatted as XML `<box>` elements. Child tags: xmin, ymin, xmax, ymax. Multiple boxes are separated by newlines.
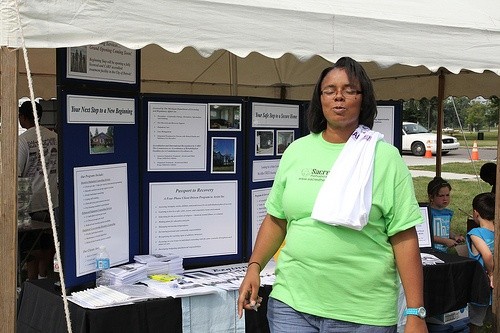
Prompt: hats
<box><xmin>428</xmin><ymin>176</ymin><xmax>450</xmax><ymax>193</ymax></box>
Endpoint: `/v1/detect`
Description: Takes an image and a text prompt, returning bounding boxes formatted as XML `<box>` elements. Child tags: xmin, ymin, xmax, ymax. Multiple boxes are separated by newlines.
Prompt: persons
<box><xmin>465</xmin><ymin>192</ymin><xmax>496</xmax><ymax>333</ymax></box>
<box><xmin>17</xmin><ymin>101</ymin><xmax>58</xmax><ymax>286</ymax></box>
<box><xmin>238</xmin><ymin>56</ymin><xmax>427</xmax><ymax>332</ymax></box>
<box><xmin>428</xmin><ymin>176</ymin><xmax>465</xmax><ymax>255</ymax></box>
<box><xmin>480</xmin><ymin>163</ymin><xmax>497</xmax><ymax>192</ymax></box>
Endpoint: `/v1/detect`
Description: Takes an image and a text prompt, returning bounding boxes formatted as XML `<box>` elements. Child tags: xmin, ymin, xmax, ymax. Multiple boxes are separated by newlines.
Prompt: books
<box><xmin>418</xmin><ymin>252</ymin><xmax>445</xmax><ymax>266</ymax></box>
<box><xmin>71</xmin><ymin>235</ymin><xmax>275</xmax><ymax>308</ymax></box>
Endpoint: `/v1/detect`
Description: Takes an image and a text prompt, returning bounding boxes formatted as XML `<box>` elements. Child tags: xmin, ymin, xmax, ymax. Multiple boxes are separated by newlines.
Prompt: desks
<box><xmin>18</xmin><ymin>221</ymin><xmax>59</xmax><ymax>287</ymax></box>
<box><xmin>18</xmin><ymin>248</ymin><xmax>492</xmax><ymax>333</ymax></box>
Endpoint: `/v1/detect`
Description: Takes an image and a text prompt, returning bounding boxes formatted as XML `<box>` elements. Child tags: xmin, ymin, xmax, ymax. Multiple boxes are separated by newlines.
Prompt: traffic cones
<box><xmin>423</xmin><ymin>140</ymin><xmax>433</xmax><ymax>159</ymax></box>
<box><xmin>469</xmin><ymin>140</ymin><xmax>480</xmax><ymax>160</ymax></box>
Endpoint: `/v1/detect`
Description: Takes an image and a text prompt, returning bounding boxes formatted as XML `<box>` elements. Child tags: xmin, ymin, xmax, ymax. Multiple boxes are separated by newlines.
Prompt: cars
<box><xmin>401</xmin><ymin>121</ymin><xmax>460</xmax><ymax>157</ymax></box>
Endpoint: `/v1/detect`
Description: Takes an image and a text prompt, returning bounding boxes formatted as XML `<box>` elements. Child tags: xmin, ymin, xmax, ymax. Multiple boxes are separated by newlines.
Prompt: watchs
<box><xmin>403</xmin><ymin>306</ymin><xmax>427</xmax><ymax>318</ymax></box>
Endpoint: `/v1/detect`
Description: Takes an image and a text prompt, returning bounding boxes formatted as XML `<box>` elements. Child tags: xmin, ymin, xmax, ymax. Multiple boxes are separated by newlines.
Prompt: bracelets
<box><xmin>248</xmin><ymin>261</ymin><xmax>261</xmax><ymax>274</ymax></box>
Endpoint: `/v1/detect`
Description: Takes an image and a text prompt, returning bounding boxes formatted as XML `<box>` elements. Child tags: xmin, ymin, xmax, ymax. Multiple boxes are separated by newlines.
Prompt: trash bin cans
<box><xmin>478</xmin><ymin>133</ymin><xmax>483</xmax><ymax>140</ymax></box>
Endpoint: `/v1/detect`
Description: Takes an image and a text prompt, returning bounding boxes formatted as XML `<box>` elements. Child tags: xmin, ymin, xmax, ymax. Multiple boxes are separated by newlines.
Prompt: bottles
<box><xmin>53</xmin><ymin>242</ymin><xmax>61</xmax><ymax>290</ymax></box>
<box><xmin>96</xmin><ymin>245</ymin><xmax>111</xmax><ymax>287</ymax></box>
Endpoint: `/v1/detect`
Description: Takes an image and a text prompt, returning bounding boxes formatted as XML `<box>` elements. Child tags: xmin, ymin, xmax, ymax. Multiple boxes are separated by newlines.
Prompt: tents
<box><xmin>0</xmin><ymin>0</ymin><xmax>499</xmax><ymax>333</ymax></box>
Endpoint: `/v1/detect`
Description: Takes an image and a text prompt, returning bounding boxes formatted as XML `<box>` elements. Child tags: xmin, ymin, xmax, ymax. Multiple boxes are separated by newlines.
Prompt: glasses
<box><xmin>321</xmin><ymin>89</ymin><xmax>362</xmax><ymax>99</ymax></box>
